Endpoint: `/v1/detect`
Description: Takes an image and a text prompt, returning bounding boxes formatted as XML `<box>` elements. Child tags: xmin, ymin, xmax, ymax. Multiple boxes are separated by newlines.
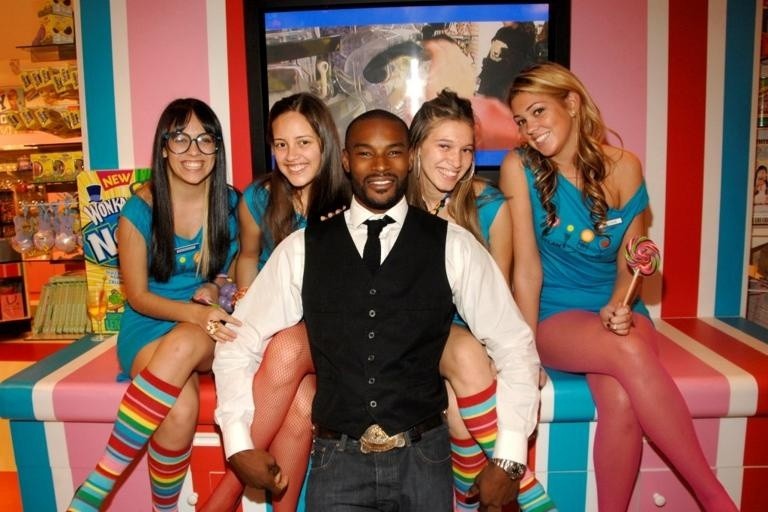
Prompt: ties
<box><xmin>363</xmin><ymin>218</ymin><xmax>387</xmax><ymax>274</ymax></box>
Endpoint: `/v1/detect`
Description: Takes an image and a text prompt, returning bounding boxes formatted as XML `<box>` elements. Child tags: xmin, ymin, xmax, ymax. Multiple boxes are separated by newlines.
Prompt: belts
<box><xmin>318</xmin><ymin>413</ymin><xmax>443</xmax><ymax>453</ymax></box>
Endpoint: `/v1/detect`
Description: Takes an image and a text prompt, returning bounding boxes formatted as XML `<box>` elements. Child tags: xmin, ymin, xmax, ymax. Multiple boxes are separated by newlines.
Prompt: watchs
<box><xmin>492</xmin><ymin>458</ymin><xmax>527</xmax><ymax>480</ymax></box>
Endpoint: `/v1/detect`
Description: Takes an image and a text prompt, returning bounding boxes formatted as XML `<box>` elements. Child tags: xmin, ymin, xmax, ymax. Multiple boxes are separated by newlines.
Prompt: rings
<box><xmin>206</xmin><ymin>319</ymin><xmax>221</xmax><ymax>335</ymax></box>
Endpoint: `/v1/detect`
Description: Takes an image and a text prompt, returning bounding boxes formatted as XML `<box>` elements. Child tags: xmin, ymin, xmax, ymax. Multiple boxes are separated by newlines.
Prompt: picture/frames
<box><xmin>243</xmin><ymin>0</ymin><xmax>572</xmax><ymax>183</ymax></box>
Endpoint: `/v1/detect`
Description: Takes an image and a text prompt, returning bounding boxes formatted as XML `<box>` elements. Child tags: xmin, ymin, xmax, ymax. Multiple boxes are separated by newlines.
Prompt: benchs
<box><xmin>0</xmin><ymin>316</ymin><xmax>768</xmax><ymax>512</ymax></box>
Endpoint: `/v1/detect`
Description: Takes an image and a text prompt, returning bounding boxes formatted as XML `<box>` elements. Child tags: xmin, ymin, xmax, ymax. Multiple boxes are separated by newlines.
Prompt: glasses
<box><xmin>164</xmin><ymin>132</ymin><xmax>220</xmax><ymax>155</ymax></box>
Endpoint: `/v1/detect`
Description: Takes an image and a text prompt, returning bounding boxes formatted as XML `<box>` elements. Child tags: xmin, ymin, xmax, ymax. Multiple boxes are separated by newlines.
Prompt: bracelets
<box><xmin>213</xmin><ymin>282</ymin><xmax>221</xmax><ymax>289</ymax></box>
<box><xmin>231</xmin><ymin>286</ymin><xmax>248</xmax><ymax>308</ymax></box>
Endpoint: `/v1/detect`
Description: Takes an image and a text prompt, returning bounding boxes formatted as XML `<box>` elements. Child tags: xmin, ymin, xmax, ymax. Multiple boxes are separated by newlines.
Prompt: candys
<box><xmin>622</xmin><ymin>235</ymin><xmax>661</xmax><ymax>306</ymax></box>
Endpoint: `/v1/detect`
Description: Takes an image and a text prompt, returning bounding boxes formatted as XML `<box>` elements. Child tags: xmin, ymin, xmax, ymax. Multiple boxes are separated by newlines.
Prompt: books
<box><xmin>746</xmin><ymin>61</ymin><xmax>768</xmax><ymax>295</ymax></box>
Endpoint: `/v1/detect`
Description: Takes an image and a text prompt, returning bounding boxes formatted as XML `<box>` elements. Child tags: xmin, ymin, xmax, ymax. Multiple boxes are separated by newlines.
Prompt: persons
<box><xmin>66</xmin><ymin>97</ymin><xmax>242</xmax><ymax>511</ymax></box>
<box><xmin>406</xmin><ymin>88</ymin><xmax>559</xmax><ymax>512</ymax></box>
<box><xmin>498</xmin><ymin>62</ymin><xmax>742</xmax><ymax>512</ymax></box>
<box><xmin>208</xmin><ymin>108</ymin><xmax>541</xmax><ymax>512</ymax></box>
<box><xmin>195</xmin><ymin>92</ymin><xmax>352</xmax><ymax>511</ymax></box>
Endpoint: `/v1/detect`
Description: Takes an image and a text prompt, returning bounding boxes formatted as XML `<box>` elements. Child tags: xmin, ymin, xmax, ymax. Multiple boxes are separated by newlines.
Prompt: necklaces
<box><xmin>421</xmin><ymin>192</ymin><xmax>452</xmax><ymax>216</ymax></box>
